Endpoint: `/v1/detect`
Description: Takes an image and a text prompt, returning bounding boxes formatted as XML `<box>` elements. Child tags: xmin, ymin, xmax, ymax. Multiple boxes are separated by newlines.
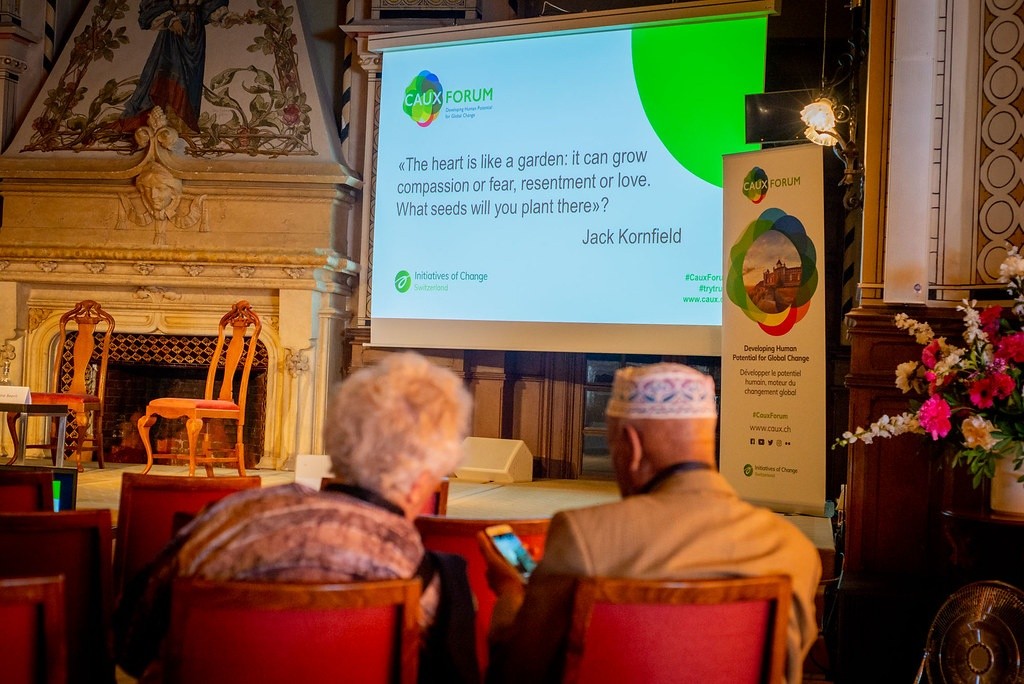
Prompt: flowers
<box><xmin>829</xmin><ymin>239</ymin><xmax>1024</xmax><ymax>486</ymax></box>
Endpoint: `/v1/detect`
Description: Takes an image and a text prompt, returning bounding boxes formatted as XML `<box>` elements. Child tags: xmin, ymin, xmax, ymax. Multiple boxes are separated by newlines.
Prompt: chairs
<box><xmin>1</xmin><ymin>300</ymin><xmax>118</xmax><ymax>476</ymax></box>
<box><xmin>136</xmin><ymin>297</ymin><xmax>264</xmax><ymax>477</ymax></box>
<box><xmin>918</xmin><ymin>577</ymin><xmax>1024</xmax><ymax>684</ymax></box>
<box><xmin>0</xmin><ymin>465</ymin><xmax>792</xmax><ymax>684</ymax></box>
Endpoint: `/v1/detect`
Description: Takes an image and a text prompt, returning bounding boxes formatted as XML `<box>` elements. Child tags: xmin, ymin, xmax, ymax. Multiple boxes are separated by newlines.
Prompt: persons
<box><xmin>116</xmin><ymin>351</ymin><xmax>474</xmax><ymax>684</ymax></box>
<box><xmin>136</xmin><ymin>162</ymin><xmax>181</xmax><ymax>219</ymax></box>
<box><xmin>475</xmin><ymin>362</ymin><xmax>823</xmax><ymax>684</ymax></box>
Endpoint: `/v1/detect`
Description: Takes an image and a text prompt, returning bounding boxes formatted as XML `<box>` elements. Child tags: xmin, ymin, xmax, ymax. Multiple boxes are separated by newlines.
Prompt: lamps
<box><xmin>451</xmin><ymin>437</ymin><xmax>533</xmax><ymax>484</ymax></box>
<box><xmin>798</xmin><ymin>98</ymin><xmax>865</xmax><ymax>211</ymax></box>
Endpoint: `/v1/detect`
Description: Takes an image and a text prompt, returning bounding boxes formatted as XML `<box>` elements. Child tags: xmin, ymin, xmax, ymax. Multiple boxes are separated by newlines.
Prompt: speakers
<box><xmin>294</xmin><ymin>455</ymin><xmax>334</xmax><ymax>491</ymax></box>
<box><xmin>453</xmin><ymin>436</ymin><xmax>534</xmax><ymax>483</ymax></box>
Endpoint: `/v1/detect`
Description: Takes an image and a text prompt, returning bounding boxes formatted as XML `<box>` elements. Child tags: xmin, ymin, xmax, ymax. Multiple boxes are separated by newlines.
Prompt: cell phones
<box><xmin>484</xmin><ymin>524</ymin><xmax>536</xmax><ymax>583</ymax></box>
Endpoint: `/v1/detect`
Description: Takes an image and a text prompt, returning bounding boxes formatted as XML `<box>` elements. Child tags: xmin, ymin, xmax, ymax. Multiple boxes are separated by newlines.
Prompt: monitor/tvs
<box><xmin>0</xmin><ymin>465</ymin><xmax>79</xmax><ymax>514</ymax></box>
<box><xmin>743</xmin><ymin>89</ymin><xmax>820</xmax><ymax>148</ymax></box>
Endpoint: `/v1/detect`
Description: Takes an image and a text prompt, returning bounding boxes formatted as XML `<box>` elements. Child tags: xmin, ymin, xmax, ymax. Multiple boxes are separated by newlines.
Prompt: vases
<box><xmin>994</xmin><ymin>427</ymin><xmax>1024</xmax><ymax>518</ymax></box>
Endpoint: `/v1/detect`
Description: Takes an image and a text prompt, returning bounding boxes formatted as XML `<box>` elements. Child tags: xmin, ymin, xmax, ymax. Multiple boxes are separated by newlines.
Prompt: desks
<box><xmin>941</xmin><ymin>496</ymin><xmax>1024</xmax><ymax>601</ymax></box>
<box><xmin>0</xmin><ymin>393</ymin><xmax>79</xmax><ymax>471</ymax></box>
